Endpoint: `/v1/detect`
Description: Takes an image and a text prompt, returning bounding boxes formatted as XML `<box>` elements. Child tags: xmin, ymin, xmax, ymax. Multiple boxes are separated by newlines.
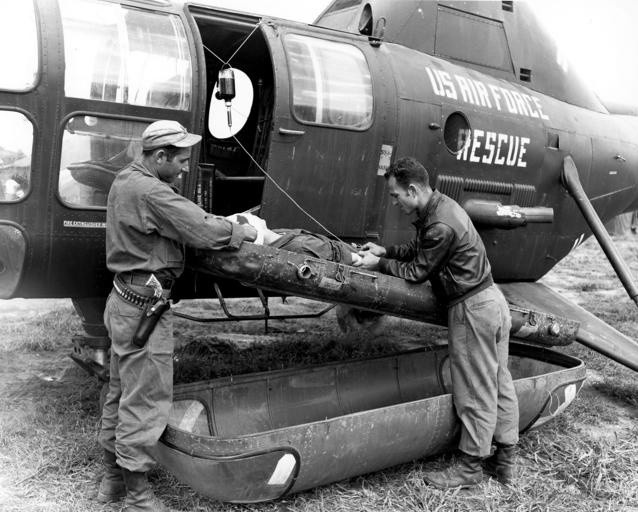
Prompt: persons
<box><xmin>94</xmin><ymin>119</ymin><xmax>258</xmax><ymax>512</ymax></box>
<box><xmin>229</xmin><ymin>211</ymin><xmax>359</xmax><ymax>266</ymax></box>
<box><xmin>351</xmin><ymin>158</ymin><xmax>518</xmax><ymax>488</ymax></box>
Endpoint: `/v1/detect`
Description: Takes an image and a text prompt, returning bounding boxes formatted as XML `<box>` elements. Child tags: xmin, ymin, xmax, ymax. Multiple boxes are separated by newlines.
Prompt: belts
<box><xmin>122</xmin><ymin>273</ymin><xmax>173</xmax><ymax>290</ymax></box>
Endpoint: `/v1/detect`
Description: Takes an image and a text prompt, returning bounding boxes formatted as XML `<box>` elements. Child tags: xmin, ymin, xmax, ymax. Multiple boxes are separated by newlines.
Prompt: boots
<box><xmin>120</xmin><ymin>468</ymin><xmax>181</xmax><ymax>512</ymax></box>
<box><xmin>485</xmin><ymin>445</ymin><xmax>518</xmax><ymax>483</ymax></box>
<box><xmin>95</xmin><ymin>448</ymin><xmax>127</xmax><ymax>504</ymax></box>
<box><xmin>423</xmin><ymin>450</ymin><xmax>484</xmax><ymax>489</ymax></box>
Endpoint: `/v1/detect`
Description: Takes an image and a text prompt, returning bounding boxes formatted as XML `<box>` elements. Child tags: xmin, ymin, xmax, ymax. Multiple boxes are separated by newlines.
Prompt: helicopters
<box><xmin>0</xmin><ymin>0</ymin><xmax>637</xmax><ymax>376</ymax></box>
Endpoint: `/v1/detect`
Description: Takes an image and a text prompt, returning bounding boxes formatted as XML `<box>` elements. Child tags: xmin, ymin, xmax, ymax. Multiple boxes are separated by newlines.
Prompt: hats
<box><xmin>141</xmin><ymin>120</ymin><xmax>201</xmax><ymax>154</ymax></box>
